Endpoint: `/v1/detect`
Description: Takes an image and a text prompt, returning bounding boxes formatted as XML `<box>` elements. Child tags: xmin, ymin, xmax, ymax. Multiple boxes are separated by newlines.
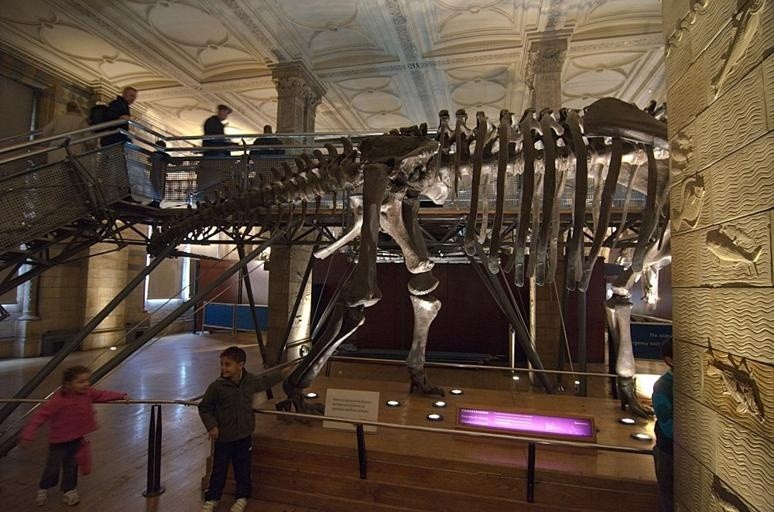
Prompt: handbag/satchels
<box><xmin>73</xmin><ymin>437</ymin><xmax>91</xmax><ymax>476</ymax></box>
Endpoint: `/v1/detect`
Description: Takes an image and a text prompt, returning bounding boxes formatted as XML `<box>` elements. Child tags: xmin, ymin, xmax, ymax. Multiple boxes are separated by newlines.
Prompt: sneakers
<box><xmin>35</xmin><ymin>489</ymin><xmax>49</xmax><ymax>506</ymax></box>
<box><xmin>146</xmin><ymin>201</ymin><xmax>160</xmax><ymax>208</ymax></box>
<box><xmin>62</xmin><ymin>488</ymin><xmax>80</xmax><ymax>506</ymax></box>
<box><xmin>122</xmin><ymin>195</ymin><xmax>141</xmax><ymax>204</ymax></box>
<box><xmin>200</xmin><ymin>500</ymin><xmax>218</xmax><ymax>512</ymax></box>
<box><xmin>229</xmin><ymin>497</ymin><xmax>248</xmax><ymax>512</ymax></box>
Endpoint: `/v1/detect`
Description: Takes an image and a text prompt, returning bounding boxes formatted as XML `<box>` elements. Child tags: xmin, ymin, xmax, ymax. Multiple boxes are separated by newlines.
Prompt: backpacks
<box><xmin>88</xmin><ymin>100</ymin><xmax>124</xmax><ymax>133</ymax></box>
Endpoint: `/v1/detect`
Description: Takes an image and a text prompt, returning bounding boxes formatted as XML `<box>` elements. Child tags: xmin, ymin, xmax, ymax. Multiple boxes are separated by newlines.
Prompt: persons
<box><xmin>41</xmin><ymin>101</ymin><xmax>97</xmax><ymax>165</ymax></box>
<box><xmin>98</xmin><ymin>84</ymin><xmax>144</xmax><ymax>205</ymax></box>
<box><xmin>147</xmin><ymin>139</ymin><xmax>183</xmax><ymax>207</ymax></box>
<box><xmin>249</xmin><ymin>124</ymin><xmax>286</xmax><ymax>155</ymax></box>
<box><xmin>18</xmin><ymin>364</ymin><xmax>133</xmax><ymax>507</ymax></box>
<box><xmin>649</xmin><ymin>336</ymin><xmax>674</xmax><ymax>512</ymax></box>
<box><xmin>202</xmin><ymin>104</ymin><xmax>235</xmax><ymax>158</ymax></box>
<box><xmin>196</xmin><ymin>347</ymin><xmax>296</xmax><ymax>512</ymax></box>
